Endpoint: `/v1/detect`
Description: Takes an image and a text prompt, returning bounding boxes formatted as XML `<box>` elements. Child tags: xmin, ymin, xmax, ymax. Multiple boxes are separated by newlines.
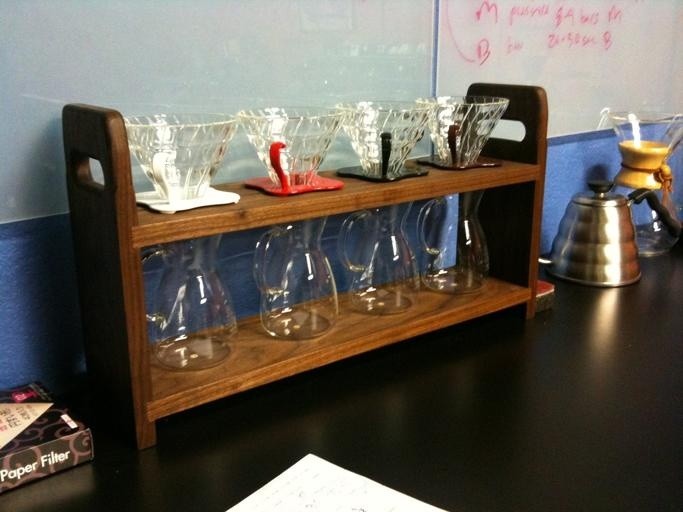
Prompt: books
<box><xmin>0</xmin><ymin>381</ymin><xmax>94</xmax><ymax>492</ymax></box>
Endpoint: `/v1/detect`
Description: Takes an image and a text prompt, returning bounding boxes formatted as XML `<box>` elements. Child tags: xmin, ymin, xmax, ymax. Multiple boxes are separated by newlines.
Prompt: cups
<box><xmin>335</xmin><ymin>100</ymin><xmax>436</xmax><ymax>178</ymax></box>
<box><xmin>237</xmin><ymin>103</ymin><xmax>347</xmax><ymax>187</ymax></box>
<box><xmin>123</xmin><ymin>114</ymin><xmax>240</xmax><ymax>205</ymax></box>
<box><xmin>415</xmin><ymin>95</ymin><xmax>510</xmax><ymax>169</ymax></box>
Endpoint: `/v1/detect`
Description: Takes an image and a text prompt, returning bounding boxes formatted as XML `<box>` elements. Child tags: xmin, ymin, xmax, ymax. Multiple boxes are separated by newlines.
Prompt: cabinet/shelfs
<box><xmin>64</xmin><ymin>82</ymin><xmax>548</xmax><ymax>452</ymax></box>
<box><xmin>0</xmin><ymin>241</ymin><xmax>683</xmax><ymax>512</ymax></box>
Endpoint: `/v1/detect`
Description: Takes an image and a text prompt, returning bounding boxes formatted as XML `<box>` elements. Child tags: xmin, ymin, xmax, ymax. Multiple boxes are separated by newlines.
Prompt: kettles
<box><xmin>548</xmin><ymin>180</ymin><xmax>680</xmax><ymax>286</ymax></box>
